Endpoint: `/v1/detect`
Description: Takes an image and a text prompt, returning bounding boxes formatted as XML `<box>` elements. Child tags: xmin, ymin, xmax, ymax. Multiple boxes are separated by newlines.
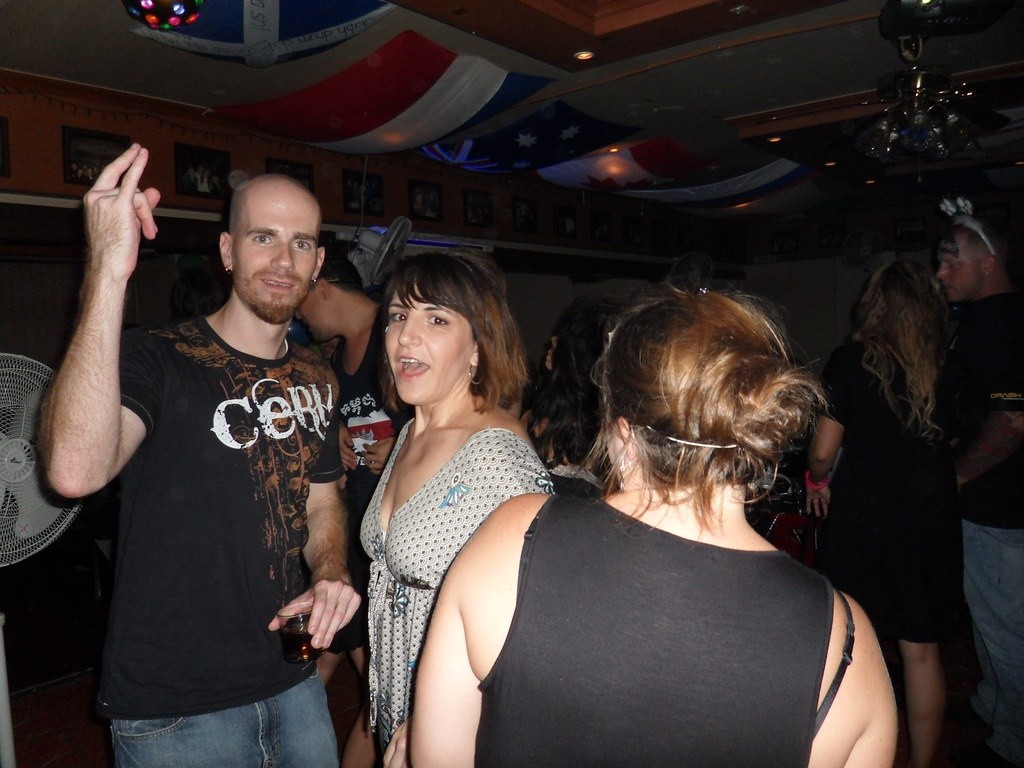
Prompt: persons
<box><xmin>36</xmin><ymin>141</ymin><xmax>1024</xmax><ymax>768</ymax></box>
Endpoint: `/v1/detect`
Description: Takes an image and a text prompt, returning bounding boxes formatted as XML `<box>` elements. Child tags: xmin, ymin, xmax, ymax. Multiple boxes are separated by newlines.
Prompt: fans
<box><xmin>335</xmin><ymin>215</ymin><xmax>413</xmax><ymax>282</ymax></box>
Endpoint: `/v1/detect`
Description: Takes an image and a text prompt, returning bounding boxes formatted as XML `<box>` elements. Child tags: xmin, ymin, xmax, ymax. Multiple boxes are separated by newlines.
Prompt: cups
<box><xmin>277</xmin><ymin>601</ymin><xmax>321</xmax><ymax>664</ymax></box>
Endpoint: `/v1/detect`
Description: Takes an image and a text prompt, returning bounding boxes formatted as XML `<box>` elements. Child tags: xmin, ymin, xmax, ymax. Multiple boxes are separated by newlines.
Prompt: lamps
<box><xmin>0</xmin><ymin>351</ymin><xmax>87</xmax><ymax>624</ymax></box>
<box><xmin>860</xmin><ymin>32</ymin><xmax>981</xmax><ymax>167</ymax></box>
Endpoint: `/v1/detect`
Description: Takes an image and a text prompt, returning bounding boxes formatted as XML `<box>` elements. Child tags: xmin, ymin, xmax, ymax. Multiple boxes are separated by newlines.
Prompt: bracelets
<box><xmin>806</xmin><ymin>470</ymin><xmax>830</xmax><ymax>491</ymax></box>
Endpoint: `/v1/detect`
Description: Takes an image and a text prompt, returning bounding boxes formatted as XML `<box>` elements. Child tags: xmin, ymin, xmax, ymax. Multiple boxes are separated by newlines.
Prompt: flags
<box><xmin>537</xmin><ymin>141</ymin><xmax>722</xmax><ymax>192</ymax></box>
<box><xmin>211</xmin><ymin>27</ymin><xmax>560</xmax><ymax>156</ymax></box>
<box><xmin>418</xmin><ymin>103</ymin><xmax>647</xmax><ymax>179</ymax></box>
<box><xmin>622</xmin><ymin>146</ymin><xmax>811</xmax><ymax>212</ymax></box>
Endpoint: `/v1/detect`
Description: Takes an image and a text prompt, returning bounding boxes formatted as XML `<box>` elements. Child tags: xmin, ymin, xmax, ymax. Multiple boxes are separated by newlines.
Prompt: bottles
<box><xmin>794</xmin><ymin>478</ymin><xmax>806</xmax><ymax>517</ymax></box>
